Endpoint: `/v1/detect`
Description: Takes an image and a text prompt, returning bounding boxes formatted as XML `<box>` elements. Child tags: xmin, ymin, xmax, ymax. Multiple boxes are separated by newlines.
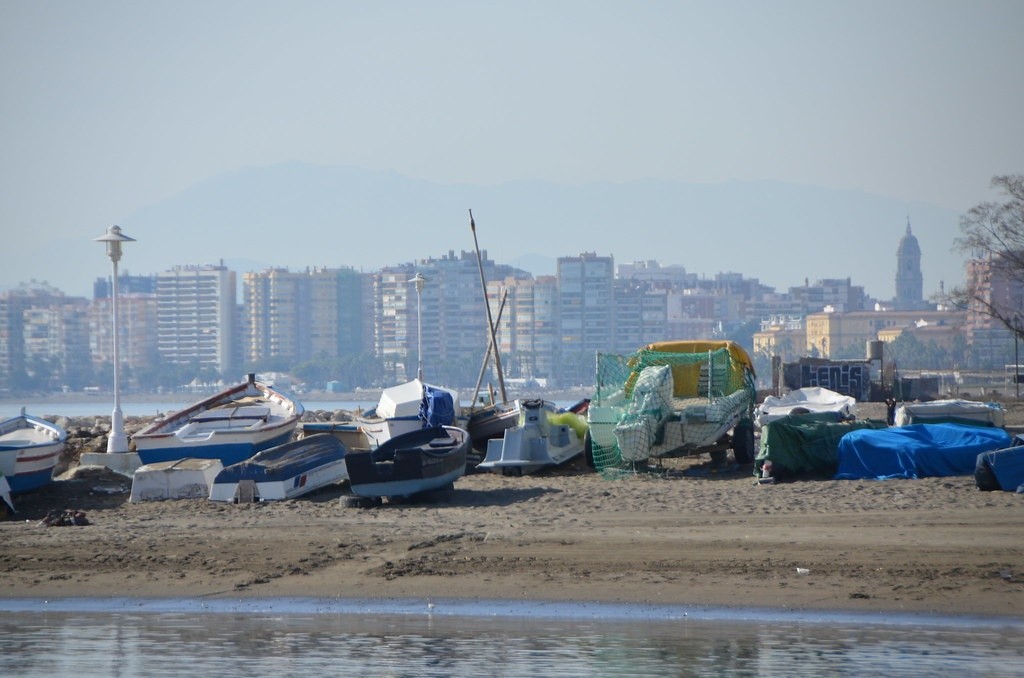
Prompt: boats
<box><xmin>895</xmin><ymin>404</ymin><xmax>1007</xmax><ymax>428</ymax></box>
<box><xmin>0</xmin><ymin>405</ymin><xmax>68</xmax><ymax>494</ymax></box>
<box><xmin>129</xmin><ymin>458</ymin><xmax>224</xmax><ymax>504</ymax></box>
<box><xmin>297</xmin><ymin>423</ymin><xmax>371</xmax><ymax>453</ymax></box>
<box><xmin>475</xmin><ymin>398</ymin><xmax>591</xmax><ymax>476</ymax></box>
<box><xmin>131</xmin><ymin>372</ymin><xmax>305</xmax><ymax>466</ymax></box>
<box><xmin>345</xmin><ymin>425</ymin><xmax>473</xmax><ymax>504</ymax></box>
<box><xmin>372</xmin><ymin>377</ymin><xmax>462</xmax><ymax>428</ymax></box>
<box><xmin>206</xmin><ymin>432</ymin><xmax>352</xmax><ymax>502</ymax></box>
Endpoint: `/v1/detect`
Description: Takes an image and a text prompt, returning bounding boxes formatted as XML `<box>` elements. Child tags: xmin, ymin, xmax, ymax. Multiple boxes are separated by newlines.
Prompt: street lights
<box><xmin>96</xmin><ymin>223</ymin><xmax>137</xmax><ymax>452</ymax></box>
<box><xmin>408</xmin><ymin>271</ymin><xmax>430</xmax><ymax>382</ymax></box>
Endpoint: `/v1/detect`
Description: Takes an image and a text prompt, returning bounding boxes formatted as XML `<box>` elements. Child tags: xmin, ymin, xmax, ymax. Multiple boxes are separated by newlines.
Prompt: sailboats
<box><xmin>463</xmin><ymin>208</ymin><xmax>558</xmax><ymax>445</ymax></box>
